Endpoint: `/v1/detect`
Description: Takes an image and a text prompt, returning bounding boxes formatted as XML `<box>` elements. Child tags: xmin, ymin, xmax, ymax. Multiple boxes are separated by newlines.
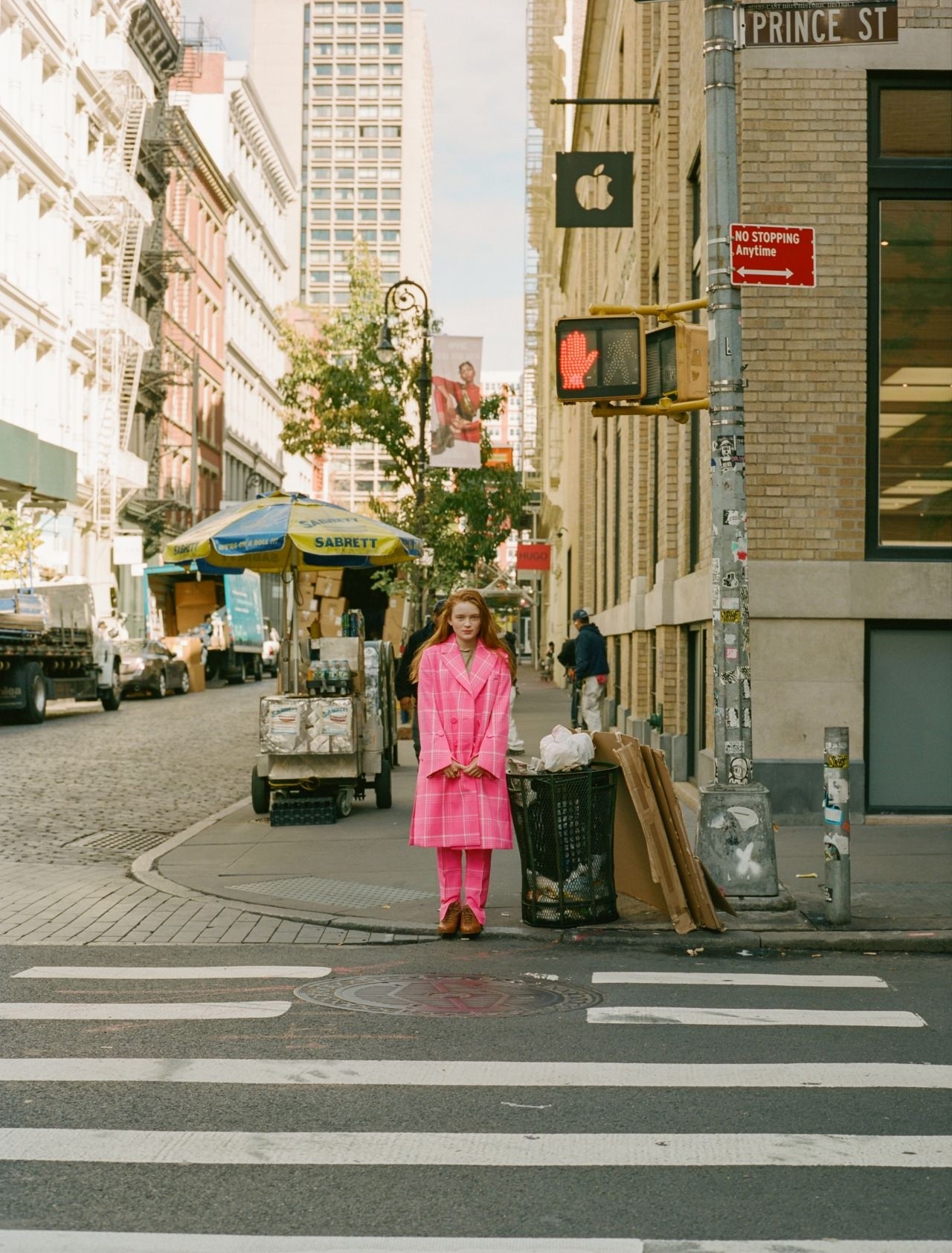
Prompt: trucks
<box><xmin>142</xmin><ymin>562</ymin><xmax>279</xmax><ymax>684</ymax></box>
<box><xmin>0</xmin><ymin>579</ymin><xmax>124</xmax><ymax>725</ymax></box>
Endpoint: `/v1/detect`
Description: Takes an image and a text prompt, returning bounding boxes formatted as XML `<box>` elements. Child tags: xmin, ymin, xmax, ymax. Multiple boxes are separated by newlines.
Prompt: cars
<box><xmin>122</xmin><ymin>637</ymin><xmax>191</xmax><ymax>700</ymax></box>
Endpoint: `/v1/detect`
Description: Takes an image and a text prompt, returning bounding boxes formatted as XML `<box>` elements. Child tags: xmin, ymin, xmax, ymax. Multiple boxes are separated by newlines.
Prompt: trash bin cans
<box><xmin>505</xmin><ymin>757</ymin><xmax>621</xmax><ymax>927</ymax></box>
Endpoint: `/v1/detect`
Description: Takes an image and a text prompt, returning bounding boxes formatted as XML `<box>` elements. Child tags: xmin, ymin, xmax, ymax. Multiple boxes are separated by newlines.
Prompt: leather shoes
<box><xmin>460</xmin><ymin>905</ymin><xmax>482</xmax><ymax>934</ymax></box>
<box><xmin>436</xmin><ymin>905</ymin><xmax>460</xmax><ymax>933</ymax></box>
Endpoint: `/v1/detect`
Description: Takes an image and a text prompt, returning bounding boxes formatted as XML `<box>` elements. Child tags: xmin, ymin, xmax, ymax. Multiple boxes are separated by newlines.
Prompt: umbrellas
<box><xmin>162</xmin><ymin>489</ymin><xmax>425</xmax><ymax>694</ymax></box>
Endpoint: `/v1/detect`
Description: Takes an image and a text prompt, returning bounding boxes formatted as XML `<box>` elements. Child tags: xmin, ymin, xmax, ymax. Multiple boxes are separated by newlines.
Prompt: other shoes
<box><xmin>505</xmin><ymin>749</ymin><xmax>524</xmax><ymax>757</ymax></box>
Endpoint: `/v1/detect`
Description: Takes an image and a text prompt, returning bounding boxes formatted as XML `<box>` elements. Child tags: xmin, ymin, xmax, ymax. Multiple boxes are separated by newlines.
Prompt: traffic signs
<box><xmin>729</xmin><ymin>223</ymin><xmax>819</xmax><ymax>289</ymax></box>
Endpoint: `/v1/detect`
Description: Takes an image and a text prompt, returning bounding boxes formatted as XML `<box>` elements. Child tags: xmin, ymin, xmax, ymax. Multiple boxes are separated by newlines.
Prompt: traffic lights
<box><xmin>639</xmin><ymin>318</ymin><xmax>709</xmax><ymax>406</ymax></box>
<box><xmin>549</xmin><ymin>310</ymin><xmax>648</xmax><ymax>406</ymax></box>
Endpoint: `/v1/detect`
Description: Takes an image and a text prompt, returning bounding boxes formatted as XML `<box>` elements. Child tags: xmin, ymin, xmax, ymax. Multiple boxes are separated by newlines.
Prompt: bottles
<box><xmin>308</xmin><ymin>659</ymin><xmax>352</xmax><ymax>698</ymax></box>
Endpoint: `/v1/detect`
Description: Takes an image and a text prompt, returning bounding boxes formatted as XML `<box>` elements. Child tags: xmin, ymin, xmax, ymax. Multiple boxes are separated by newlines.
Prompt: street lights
<box><xmin>373</xmin><ymin>276</ymin><xmax>431</xmax><ymax>632</ymax></box>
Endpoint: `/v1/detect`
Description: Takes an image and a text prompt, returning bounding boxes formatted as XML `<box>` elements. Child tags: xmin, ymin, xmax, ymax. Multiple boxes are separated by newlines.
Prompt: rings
<box><xmin>454</xmin><ymin>770</ymin><xmax>458</xmax><ymax>774</ymax></box>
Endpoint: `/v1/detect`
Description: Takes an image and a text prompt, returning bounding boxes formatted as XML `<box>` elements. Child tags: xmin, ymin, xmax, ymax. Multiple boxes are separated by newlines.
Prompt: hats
<box><xmin>572</xmin><ymin>609</ymin><xmax>588</xmax><ymax>620</ymax></box>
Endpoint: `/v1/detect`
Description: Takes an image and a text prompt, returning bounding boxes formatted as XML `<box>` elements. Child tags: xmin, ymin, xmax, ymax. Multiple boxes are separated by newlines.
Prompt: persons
<box><xmin>432</xmin><ymin>361</ymin><xmax>481</xmax><ymax>453</ymax></box>
<box><xmin>395</xmin><ymin>598</ymin><xmax>447</xmax><ymax>759</ymax></box>
<box><xmin>408</xmin><ymin>588</ymin><xmax>512</xmax><ymax>934</ymax></box>
<box><xmin>557</xmin><ymin>609</ymin><xmax>609</xmax><ymax>733</ymax></box>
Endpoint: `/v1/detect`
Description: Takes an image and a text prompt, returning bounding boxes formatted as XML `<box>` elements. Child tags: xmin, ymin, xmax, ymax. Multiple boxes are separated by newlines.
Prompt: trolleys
<box><xmin>249</xmin><ymin>631</ymin><xmax>396</xmax><ymax>819</ymax></box>
